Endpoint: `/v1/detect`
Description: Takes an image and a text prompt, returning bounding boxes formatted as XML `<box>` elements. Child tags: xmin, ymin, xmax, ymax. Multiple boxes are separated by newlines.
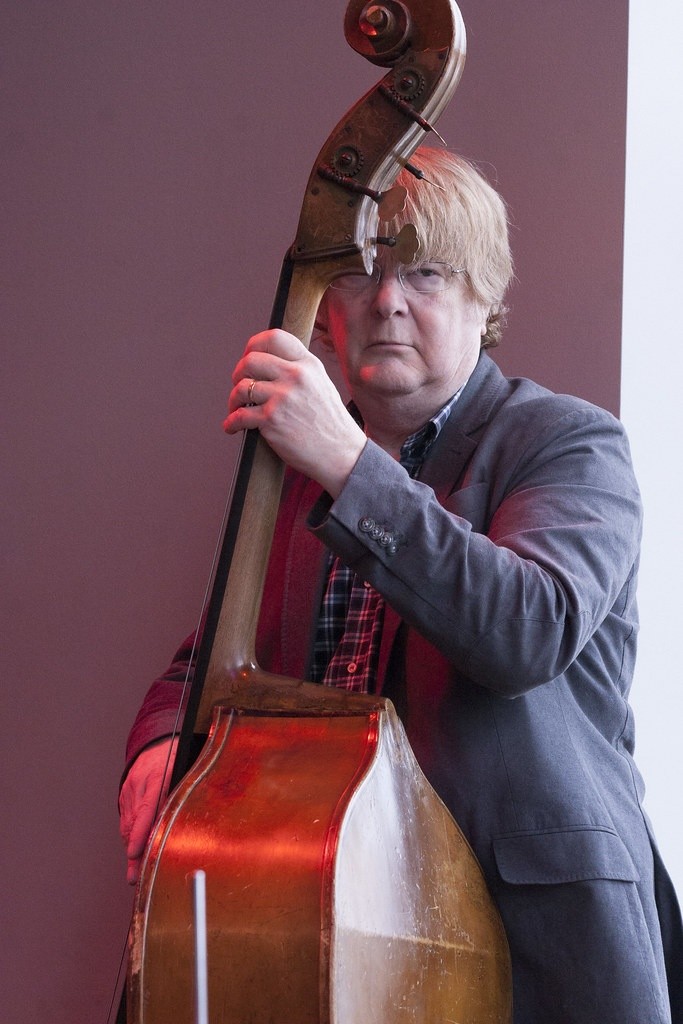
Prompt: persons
<box><xmin>119</xmin><ymin>146</ymin><xmax>683</xmax><ymax>1024</ymax></box>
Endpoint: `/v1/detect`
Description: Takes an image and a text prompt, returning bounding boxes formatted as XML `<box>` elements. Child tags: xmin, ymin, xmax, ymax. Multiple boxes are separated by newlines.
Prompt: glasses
<box><xmin>329</xmin><ymin>261</ymin><xmax>466</xmax><ymax>292</ymax></box>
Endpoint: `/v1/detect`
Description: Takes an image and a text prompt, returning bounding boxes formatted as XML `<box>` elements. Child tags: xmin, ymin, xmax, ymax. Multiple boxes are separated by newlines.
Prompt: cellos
<box><xmin>114</xmin><ymin>2</ymin><xmax>521</xmax><ymax>1024</ymax></box>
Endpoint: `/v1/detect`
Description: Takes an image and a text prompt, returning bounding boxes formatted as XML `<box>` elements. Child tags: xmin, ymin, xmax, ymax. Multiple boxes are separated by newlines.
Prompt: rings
<box><xmin>248</xmin><ymin>378</ymin><xmax>259</xmax><ymax>405</ymax></box>
